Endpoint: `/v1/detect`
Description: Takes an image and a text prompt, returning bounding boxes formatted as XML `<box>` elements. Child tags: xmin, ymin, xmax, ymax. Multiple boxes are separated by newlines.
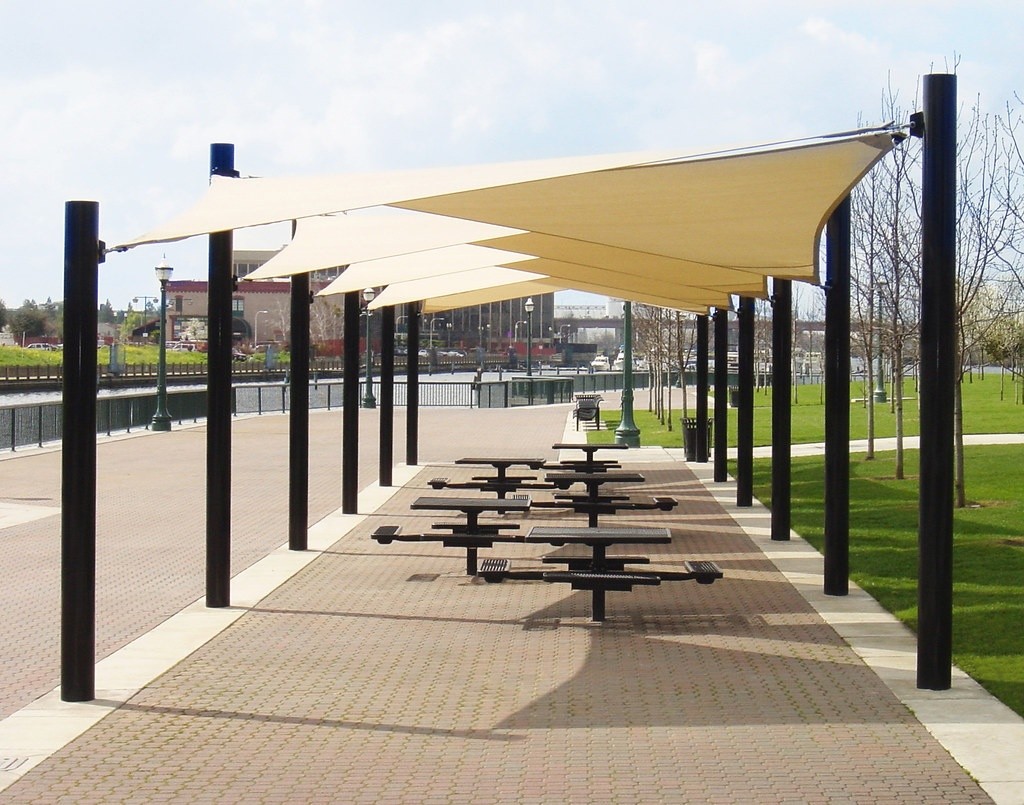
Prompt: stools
<box><xmin>374</xmin><ymin>442</ymin><xmax>723</xmax><ymax>621</ymax></box>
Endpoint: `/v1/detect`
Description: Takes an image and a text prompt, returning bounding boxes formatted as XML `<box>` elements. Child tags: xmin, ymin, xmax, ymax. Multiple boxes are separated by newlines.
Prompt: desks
<box><xmin>552</xmin><ymin>443</ymin><xmax>628</xmax><ymax>491</ymax></box>
<box><xmin>545</xmin><ymin>472</ymin><xmax>645</xmax><ymax>527</ymax></box>
<box><xmin>410</xmin><ymin>496</ymin><xmax>531</xmax><ymax>575</ymax></box>
<box><xmin>525</xmin><ymin>526</ymin><xmax>672</xmax><ymax>621</ymax></box>
<box><xmin>455</xmin><ymin>456</ymin><xmax>545</xmax><ymax>513</ymax></box>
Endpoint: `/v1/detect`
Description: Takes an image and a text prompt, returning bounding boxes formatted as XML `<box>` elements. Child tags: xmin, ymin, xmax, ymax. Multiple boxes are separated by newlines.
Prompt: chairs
<box><xmin>573</xmin><ymin>394</ymin><xmax>604</xmax><ymax>430</ymax></box>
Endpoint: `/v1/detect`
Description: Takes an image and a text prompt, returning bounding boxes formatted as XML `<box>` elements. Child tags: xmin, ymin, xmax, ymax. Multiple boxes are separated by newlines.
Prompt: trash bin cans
<box><xmin>679</xmin><ymin>417</ymin><xmax>713</xmax><ymax>462</ymax></box>
<box><xmin>729</xmin><ymin>385</ymin><xmax>739</xmax><ymax>407</ymax></box>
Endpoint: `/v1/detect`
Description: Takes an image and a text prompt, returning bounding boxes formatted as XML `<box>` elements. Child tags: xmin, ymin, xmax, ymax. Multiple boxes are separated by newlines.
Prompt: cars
<box><xmin>172</xmin><ymin>343</ymin><xmax>196</xmax><ymax>353</ymax></box>
<box><xmin>231</xmin><ymin>346</ymin><xmax>247</xmax><ymax>362</ymax></box>
<box><xmin>591</xmin><ymin>356</ymin><xmax>610</xmax><ymax>372</ymax></box>
<box><xmin>25</xmin><ymin>343</ymin><xmax>54</xmax><ymax>352</ymax></box>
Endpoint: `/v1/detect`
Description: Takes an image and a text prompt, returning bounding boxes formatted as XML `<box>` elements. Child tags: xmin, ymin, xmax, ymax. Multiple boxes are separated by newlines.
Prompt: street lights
<box><xmin>523</xmin><ymin>298</ymin><xmax>535</xmax><ymax>376</ymax></box>
<box><xmin>427</xmin><ymin>318</ymin><xmax>445</xmax><ymax>376</ymax></box>
<box><xmin>361</xmin><ymin>286</ymin><xmax>376</xmax><ymax>407</ymax></box>
<box><xmin>252</xmin><ymin>310</ymin><xmax>269</xmax><ymax>352</ymax></box>
<box><xmin>513</xmin><ymin>321</ymin><xmax>527</xmax><ymax>343</ymax></box>
<box><xmin>870</xmin><ymin>278</ymin><xmax>888</xmax><ymax>402</ymax></box>
<box><xmin>133</xmin><ymin>295</ymin><xmax>158</xmax><ymax>345</ymax></box>
<box><xmin>560</xmin><ymin>323</ymin><xmax>571</xmax><ymax>344</ymax></box>
<box><xmin>396</xmin><ymin>315</ymin><xmax>409</xmax><ymax>335</ymax></box>
<box><xmin>150</xmin><ymin>252</ymin><xmax>175</xmax><ymax>431</ymax></box>
